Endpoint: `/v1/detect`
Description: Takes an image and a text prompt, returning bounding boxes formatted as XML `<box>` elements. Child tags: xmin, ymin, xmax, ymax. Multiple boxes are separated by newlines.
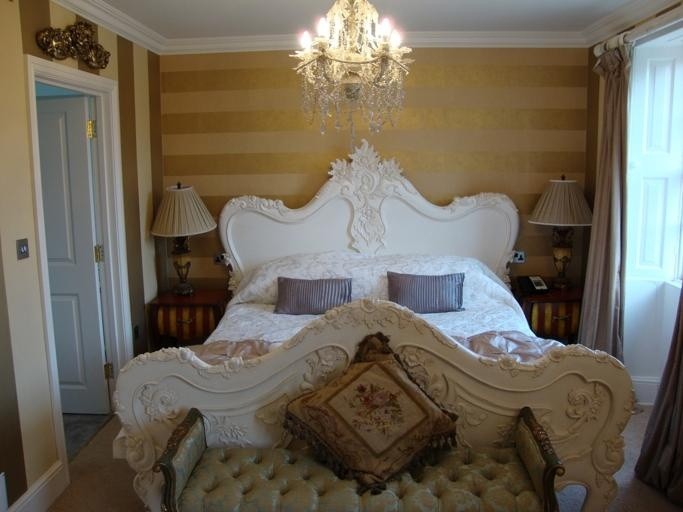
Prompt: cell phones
<box><xmin>528</xmin><ymin>275</ymin><xmax>549</xmax><ymax>294</ymax></box>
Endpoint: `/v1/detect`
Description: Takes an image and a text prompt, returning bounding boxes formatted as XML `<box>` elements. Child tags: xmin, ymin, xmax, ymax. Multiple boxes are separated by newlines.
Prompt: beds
<box><xmin>111</xmin><ymin>139</ymin><xmax>635</xmax><ymax>512</ymax></box>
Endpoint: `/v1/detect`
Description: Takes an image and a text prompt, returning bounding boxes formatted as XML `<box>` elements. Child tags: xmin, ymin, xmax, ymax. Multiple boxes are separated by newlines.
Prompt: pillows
<box><xmin>387</xmin><ymin>271</ymin><xmax>466</xmax><ymax>314</ymax></box>
<box><xmin>283</xmin><ymin>332</ymin><xmax>456</xmax><ymax>495</ymax></box>
<box><xmin>274</xmin><ymin>276</ymin><xmax>351</xmax><ymax>315</ymax></box>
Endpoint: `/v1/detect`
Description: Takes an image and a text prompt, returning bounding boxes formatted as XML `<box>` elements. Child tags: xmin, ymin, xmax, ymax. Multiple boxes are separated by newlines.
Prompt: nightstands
<box><xmin>510</xmin><ymin>276</ymin><xmax>582</xmax><ymax>345</ymax></box>
<box><xmin>149</xmin><ymin>289</ymin><xmax>226</xmax><ymax>353</ymax></box>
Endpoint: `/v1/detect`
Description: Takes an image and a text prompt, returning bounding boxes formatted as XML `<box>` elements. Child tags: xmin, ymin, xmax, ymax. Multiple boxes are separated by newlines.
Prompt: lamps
<box><xmin>288</xmin><ymin>0</ymin><xmax>414</xmax><ymax>142</ymax></box>
<box><xmin>151</xmin><ymin>181</ymin><xmax>217</xmax><ymax>294</ymax></box>
<box><xmin>528</xmin><ymin>175</ymin><xmax>592</xmax><ymax>289</ymax></box>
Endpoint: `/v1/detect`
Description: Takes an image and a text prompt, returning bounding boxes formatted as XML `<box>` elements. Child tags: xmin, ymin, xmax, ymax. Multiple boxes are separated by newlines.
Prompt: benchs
<box><xmin>152</xmin><ymin>406</ymin><xmax>565</xmax><ymax>512</ymax></box>
